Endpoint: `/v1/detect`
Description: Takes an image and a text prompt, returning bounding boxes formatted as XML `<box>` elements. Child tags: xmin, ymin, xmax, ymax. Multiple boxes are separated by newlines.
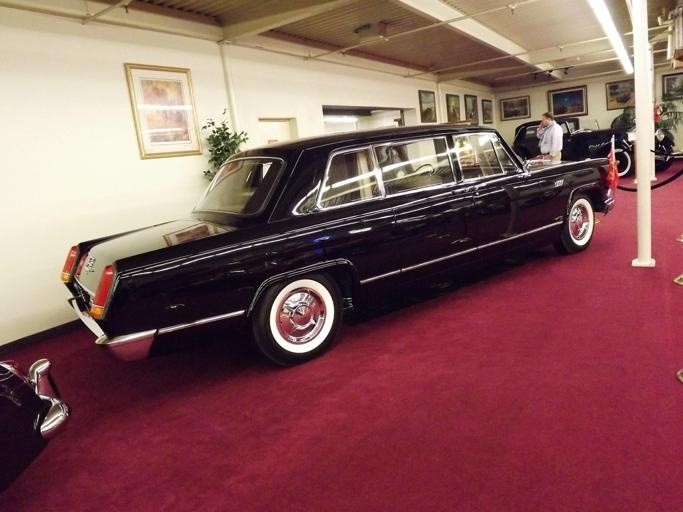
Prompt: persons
<box><xmin>536</xmin><ymin>112</ymin><xmax>564</xmax><ymax>162</ymax></box>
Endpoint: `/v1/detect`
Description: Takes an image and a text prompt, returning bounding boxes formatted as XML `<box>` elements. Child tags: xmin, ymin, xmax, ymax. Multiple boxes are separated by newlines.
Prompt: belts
<box><xmin>540</xmin><ymin>152</ymin><xmax>550</xmax><ymax>158</ymax></box>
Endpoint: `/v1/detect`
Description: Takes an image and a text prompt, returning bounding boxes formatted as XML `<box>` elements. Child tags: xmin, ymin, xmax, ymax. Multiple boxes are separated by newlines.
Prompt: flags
<box><xmin>605</xmin><ymin>137</ymin><xmax>620</xmax><ymax>192</ymax></box>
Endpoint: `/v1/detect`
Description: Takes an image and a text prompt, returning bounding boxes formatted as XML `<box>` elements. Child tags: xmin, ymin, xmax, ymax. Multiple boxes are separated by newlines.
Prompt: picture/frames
<box><xmin>546</xmin><ymin>83</ymin><xmax>588</xmax><ymax>119</ymax></box>
<box><xmin>123</xmin><ymin>62</ymin><xmax>203</xmax><ymax>160</ymax></box>
<box><xmin>418</xmin><ymin>89</ymin><xmax>493</xmax><ymax>124</ymax></box>
<box><xmin>498</xmin><ymin>94</ymin><xmax>530</xmax><ymax>121</ymax></box>
<box><xmin>605</xmin><ymin>78</ymin><xmax>635</xmax><ymax>112</ymax></box>
<box><xmin>660</xmin><ymin>71</ymin><xmax>683</xmax><ymax>101</ymax></box>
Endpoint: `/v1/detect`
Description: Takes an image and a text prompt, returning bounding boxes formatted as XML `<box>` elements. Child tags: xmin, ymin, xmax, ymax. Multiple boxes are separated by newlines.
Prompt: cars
<box><xmin>510</xmin><ymin>117</ymin><xmax>677</xmax><ymax>179</ymax></box>
<box><xmin>59</xmin><ymin>123</ymin><xmax>613</xmax><ymax>364</ymax></box>
<box><xmin>0</xmin><ymin>354</ymin><xmax>72</xmax><ymax>498</ymax></box>
